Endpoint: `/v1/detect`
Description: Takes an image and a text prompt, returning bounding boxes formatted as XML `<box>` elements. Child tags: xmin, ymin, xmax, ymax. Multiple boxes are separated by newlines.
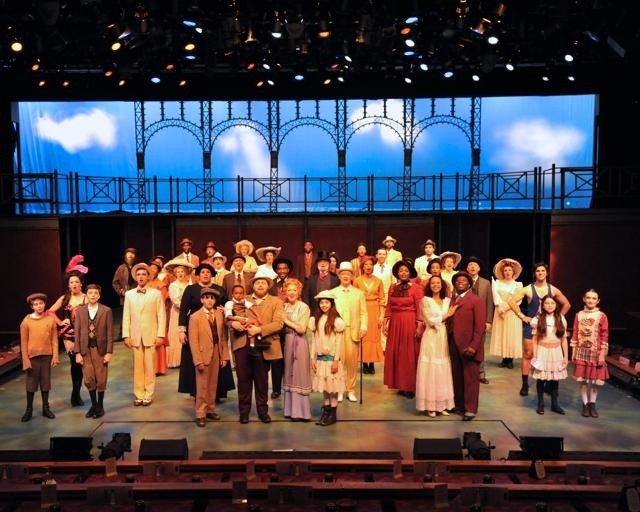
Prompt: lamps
<box><xmin>455</xmin><ymin>0</ymin><xmax>469</xmax><ymax>18</ymax></box>
<box><xmin>271</xmin><ymin>22</ymin><xmax>282</xmax><ymax>38</ymax></box>
<box><xmin>319</xmin><ymin>17</ymin><xmax>330</xmax><ymax>39</ymax></box>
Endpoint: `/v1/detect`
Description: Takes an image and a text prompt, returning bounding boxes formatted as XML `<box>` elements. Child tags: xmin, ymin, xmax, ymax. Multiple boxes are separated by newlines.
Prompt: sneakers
<box><xmin>498</xmin><ymin>358</ymin><xmax>514</xmax><ymax>369</ymax></box>
<box><xmin>520</xmin><ymin>384</ymin><xmax>564</xmax><ymax>415</ymax></box>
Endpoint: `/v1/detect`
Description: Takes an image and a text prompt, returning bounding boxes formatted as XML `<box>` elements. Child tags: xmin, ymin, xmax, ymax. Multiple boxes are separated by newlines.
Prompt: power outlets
<box><xmin>472</xmin><ymin>20</ymin><xmax>484</xmax><ymax>35</ymax></box>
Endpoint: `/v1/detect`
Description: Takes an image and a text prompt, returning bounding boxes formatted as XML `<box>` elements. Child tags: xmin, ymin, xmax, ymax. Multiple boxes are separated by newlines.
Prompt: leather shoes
<box><xmin>398</xmin><ymin>378</ymin><xmax>489</xmax><ymax>421</ymax></box>
<box><xmin>86</xmin><ymin>407</ymin><xmax>105</xmax><ymax>418</ymax></box>
<box><xmin>133</xmin><ymin>398</ymin><xmax>152</xmax><ymax>406</ymax></box>
<box><xmin>315</xmin><ymin>365</ymin><xmax>374</xmax><ymax>426</ymax></box>
<box><xmin>196</xmin><ymin>391</ymin><xmax>278</xmax><ymax>427</ymax></box>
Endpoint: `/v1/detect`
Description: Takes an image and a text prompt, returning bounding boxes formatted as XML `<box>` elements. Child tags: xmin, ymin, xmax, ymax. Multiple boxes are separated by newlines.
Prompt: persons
<box><xmin>531</xmin><ymin>295</ymin><xmax>569</xmax><ymax>414</ymax></box>
<box><xmin>188</xmin><ymin>289</ymin><xmax>230</xmax><ymax>427</ymax></box>
<box><xmin>143</xmin><ymin>261</ymin><xmax>170</xmax><ymax>377</ymax></box>
<box><xmin>490</xmin><ymin>258</ymin><xmax>524</xmax><ymax>369</ymax></box>
<box><xmin>570</xmin><ymin>289</ymin><xmax>609</xmax><ymax>418</ymax></box>
<box><xmin>224</xmin><ymin>284</ymin><xmax>273</xmax><ymax>357</ymax></box>
<box><xmin>20</xmin><ymin>294</ymin><xmax>60</xmax><ymax>422</ymax></box>
<box><xmin>48</xmin><ymin>255</ymin><xmax>90</xmax><ymax>408</ymax></box>
<box><xmin>295</xmin><ymin>237</ymin><xmax>494</xmax><ymax>403</ymax></box>
<box><xmin>227</xmin><ymin>274</ymin><xmax>285</xmax><ymax>424</ymax></box>
<box><xmin>509</xmin><ymin>262</ymin><xmax>572</xmax><ymax>396</ymax></box>
<box><xmin>73</xmin><ymin>283</ymin><xmax>114</xmax><ymax>418</ymax></box>
<box><xmin>309</xmin><ymin>289</ymin><xmax>346</xmax><ymax>426</ymax></box>
<box><xmin>112</xmin><ymin>249</ymin><xmax>138</xmax><ymax>342</ymax></box>
<box><xmin>121</xmin><ymin>263</ymin><xmax>167</xmax><ymax>406</ymax></box>
<box><xmin>280</xmin><ymin>278</ymin><xmax>313</xmax><ymax>421</ymax></box>
<box><xmin>145</xmin><ymin>237</ymin><xmax>293</xmax><ymax>405</ymax></box>
<box><xmin>415</xmin><ymin>275</ymin><xmax>460</xmax><ymax>418</ymax></box>
<box><xmin>444</xmin><ymin>271</ymin><xmax>487</xmax><ymax>421</ymax></box>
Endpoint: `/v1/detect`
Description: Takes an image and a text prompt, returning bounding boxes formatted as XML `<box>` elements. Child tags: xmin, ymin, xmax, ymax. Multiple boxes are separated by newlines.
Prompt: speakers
<box><xmin>139</xmin><ymin>439</ymin><xmax>188</xmax><ymax>460</ymax></box>
<box><xmin>413</xmin><ymin>438</ymin><xmax>463</xmax><ymax>460</ymax></box>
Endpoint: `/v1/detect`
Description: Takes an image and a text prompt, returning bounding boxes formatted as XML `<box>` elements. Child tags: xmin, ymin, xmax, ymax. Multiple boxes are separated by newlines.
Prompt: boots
<box><xmin>21</xmin><ymin>391</ymin><xmax>55</xmax><ymax>422</ymax></box>
<box><xmin>582</xmin><ymin>402</ymin><xmax>598</xmax><ymax>417</ymax></box>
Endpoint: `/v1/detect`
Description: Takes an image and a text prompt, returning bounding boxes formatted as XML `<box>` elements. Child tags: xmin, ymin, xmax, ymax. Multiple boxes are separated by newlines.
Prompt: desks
<box><xmin>1</xmin><ymin>460</ymin><xmax>640</xmax><ymax>512</ymax></box>
<box><xmin>606</xmin><ymin>353</ymin><xmax>640</xmax><ymax>400</ymax></box>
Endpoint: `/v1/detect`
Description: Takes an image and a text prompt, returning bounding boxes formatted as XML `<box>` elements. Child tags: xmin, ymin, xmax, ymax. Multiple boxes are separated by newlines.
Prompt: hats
<box><xmin>314</xmin><ymin>235</ymin><xmax>417</xmax><ymax>299</ymax></box>
<box><xmin>493</xmin><ymin>259</ymin><xmax>524</xmax><ymax>280</ymax></box>
<box><xmin>423</xmin><ymin>240</ymin><xmax>481</xmax><ymax>288</ymax></box>
<box><xmin>25</xmin><ymin>293</ymin><xmax>47</xmax><ymax>305</ymax></box>
<box><xmin>126</xmin><ymin>238</ymin><xmax>293</xmax><ymax>297</ymax></box>
